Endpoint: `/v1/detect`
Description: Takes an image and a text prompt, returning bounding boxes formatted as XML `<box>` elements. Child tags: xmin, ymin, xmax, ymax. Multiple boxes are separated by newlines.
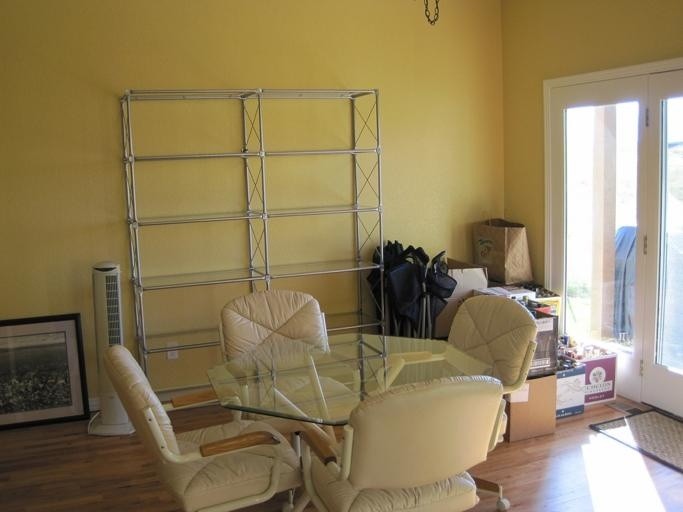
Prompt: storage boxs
<box><xmin>436</xmin><ymin>220</ymin><xmax>616</xmax><ymax>445</ymax></box>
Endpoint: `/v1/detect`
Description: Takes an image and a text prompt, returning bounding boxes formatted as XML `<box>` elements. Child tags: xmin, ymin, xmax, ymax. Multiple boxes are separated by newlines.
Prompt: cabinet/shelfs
<box><xmin>119</xmin><ymin>86</ymin><xmax>384</xmax><ymax>412</ymax></box>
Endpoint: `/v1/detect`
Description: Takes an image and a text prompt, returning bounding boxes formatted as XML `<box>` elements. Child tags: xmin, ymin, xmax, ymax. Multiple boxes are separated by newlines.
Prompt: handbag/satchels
<box><xmin>474</xmin><ymin>218</ymin><xmax>534</xmax><ymax>285</ymax></box>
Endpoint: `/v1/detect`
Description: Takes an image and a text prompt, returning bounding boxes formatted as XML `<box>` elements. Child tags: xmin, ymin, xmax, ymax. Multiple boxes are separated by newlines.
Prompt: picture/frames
<box><xmin>0</xmin><ymin>312</ymin><xmax>91</xmax><ymax>429</ymax></box>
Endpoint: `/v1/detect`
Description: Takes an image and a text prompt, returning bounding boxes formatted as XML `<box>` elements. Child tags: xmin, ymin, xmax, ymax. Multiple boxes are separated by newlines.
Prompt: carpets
<box><xmin>589</xmin><ymin>409</ymin><xmax>683</xmax><ymax>482</ymax></box>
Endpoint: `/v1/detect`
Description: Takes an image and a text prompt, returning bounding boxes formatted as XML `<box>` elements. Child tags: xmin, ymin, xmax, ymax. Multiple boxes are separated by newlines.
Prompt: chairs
<box><xmin>378</xmin><ymin>291</ymin><xmax>540</xmax><ymax>512</ymax></box>
<box><xmin>302</xmin><ymin>370</ymin><xmax>508</xmax><ymax>512</ymax></box>
<box><xmin>99</xmin><ymin>337</ymin><xmax>301</xmax><ymax>512</ymax></box>
<box><xmin>221</xmin><ymin>291</ymin><xmax>363</xmax><ymax>418</ymax></box>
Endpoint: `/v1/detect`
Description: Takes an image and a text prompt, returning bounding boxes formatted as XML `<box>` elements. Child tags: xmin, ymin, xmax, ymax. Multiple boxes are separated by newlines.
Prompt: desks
<box><xmin>202</xmin><ymin>330</ymin><xmax>501</xmax><ymax>430</ymax></box>
